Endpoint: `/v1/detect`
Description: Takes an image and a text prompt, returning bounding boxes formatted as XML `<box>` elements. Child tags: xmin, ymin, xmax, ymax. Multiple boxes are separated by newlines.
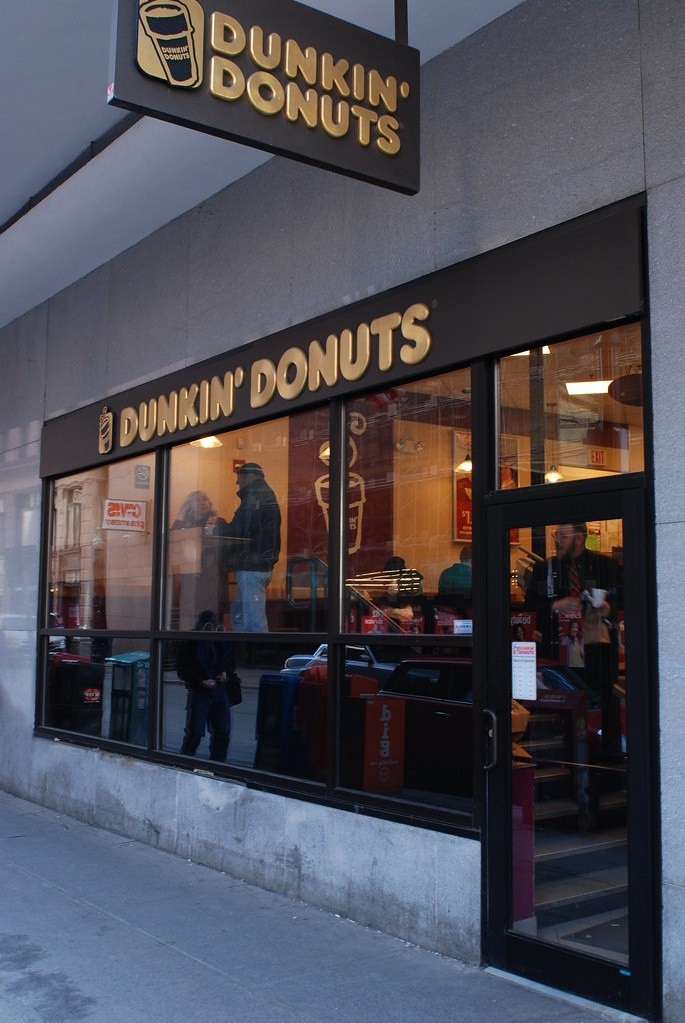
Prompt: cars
<box><xmin>278</xmin><ymin>642</ymin><xmax>626</xmax><ymax>745</ymax></box>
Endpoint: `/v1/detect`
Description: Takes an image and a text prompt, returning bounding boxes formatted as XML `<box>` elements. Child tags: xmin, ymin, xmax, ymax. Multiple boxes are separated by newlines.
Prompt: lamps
<box><xmin>454</xmin><ymin>388</ymin><xmax>472</xmax><ymax>473</ymax></box>
<box><xmin>546</xmin><ymin>402</ymin><xmax>564</xmax><ymax>482</ymax></box>
<box><xmin>318</xmin><ymin>447</ymin><xmax>331</xmax><ymax>459</ymax></box>
<box><xmin>190</xmin><ymin>436</ymin><xmax>223</xmax><ymax>448</ymax></box>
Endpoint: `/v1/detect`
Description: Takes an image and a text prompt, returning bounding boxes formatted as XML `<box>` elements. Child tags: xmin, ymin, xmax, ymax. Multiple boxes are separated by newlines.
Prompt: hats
<box><xmin>236</xmin><ymin>462</ymin><xmax>265</xmax><ymax>478</ymax></box>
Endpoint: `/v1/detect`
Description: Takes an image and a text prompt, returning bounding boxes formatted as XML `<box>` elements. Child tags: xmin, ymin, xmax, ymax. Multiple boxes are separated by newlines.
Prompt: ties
<box><xmin>567</xmin><ymin>559</ymin><xmax>581</xmax><ymax>599</ymax></box>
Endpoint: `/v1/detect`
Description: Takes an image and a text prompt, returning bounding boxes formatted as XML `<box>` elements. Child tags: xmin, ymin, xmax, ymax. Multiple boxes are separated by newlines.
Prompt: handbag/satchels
<box><xmin>224</xmin><ymin>672</ymin><xmax>242</xmax><ymax>706</ymax></box>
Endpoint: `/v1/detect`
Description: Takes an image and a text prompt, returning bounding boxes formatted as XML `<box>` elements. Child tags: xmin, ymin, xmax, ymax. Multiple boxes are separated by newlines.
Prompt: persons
<box><xmin>176</xmin><ymin>610</ymin><xmax>237</xmax><ymax>762</ymax></box>
<box><xmin>438</xmin><ymin>544</ymin><xmax>472</xmax><ymax>593</ymax></box>
<box><xmin>525</xmin><ymin>522</ymin><xmax>628</xmax><ymax>805</ymax></box>
<box><xmin>513</xmin><ymin>623</ymin><xmax>525</xmax><ymax>642</ymax></box>
<box><xmin>204</xmin><ymin>462</ymin><xmax>282</xmax><ymax>633</ymax></box>
<box><xmin>170</xmin><ymin>491</ymin><xmax>230</xmax><ymax>631</ymax></box>
<box><xmin>561</xmin><ymin>619</ymin><xmax>585</xmax><ymax>667</ymax></box>
<box><xmin>385</xmin><ymin>556</ymin><xmax>406</xmax><ymax>578</ymax></box>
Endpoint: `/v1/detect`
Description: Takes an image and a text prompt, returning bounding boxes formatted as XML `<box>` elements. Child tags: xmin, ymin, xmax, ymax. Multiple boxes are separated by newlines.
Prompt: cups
<box><xmin>592</xmin><ymin>588</ymin><xmax>608</xmax><ymax>608</ymax></box>
